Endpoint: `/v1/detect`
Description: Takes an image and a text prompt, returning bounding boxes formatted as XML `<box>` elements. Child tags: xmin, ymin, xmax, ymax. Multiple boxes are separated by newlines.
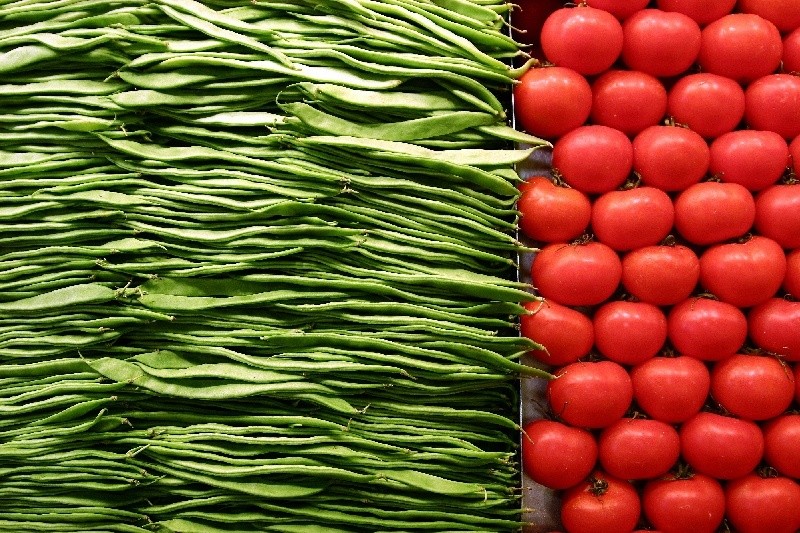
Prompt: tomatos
<box><xmin>511</xmin><ymin>0</ymin><xmax>800</xmax><ymax>533</ymax></box>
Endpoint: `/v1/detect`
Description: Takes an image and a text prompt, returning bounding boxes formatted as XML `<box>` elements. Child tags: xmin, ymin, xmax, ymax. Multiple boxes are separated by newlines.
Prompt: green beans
<box><xmin>0</xmin><ymin>0</ymin><xmax>555</xmax><ymax>533</ymax></box>
